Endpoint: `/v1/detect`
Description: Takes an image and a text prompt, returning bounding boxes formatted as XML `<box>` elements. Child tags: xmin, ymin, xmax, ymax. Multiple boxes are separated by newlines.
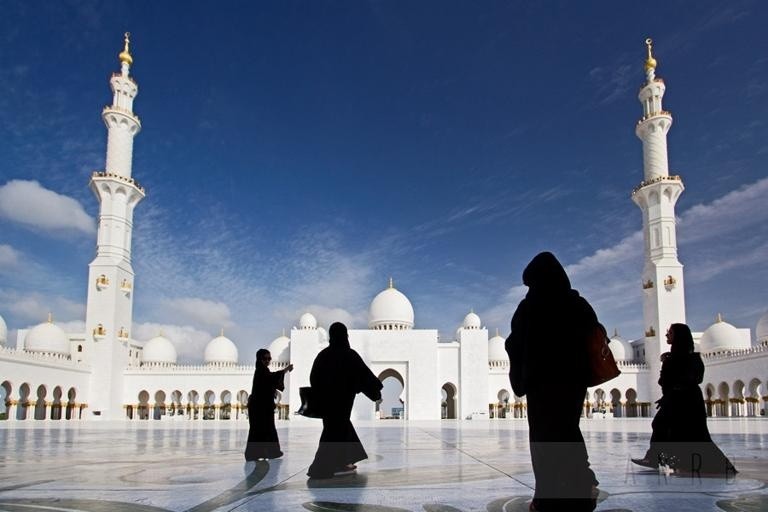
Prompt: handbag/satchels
<box><xmin>297</xmin><ymin>386</ymin><xmax>323</xmax><ymax>419</ymax></box>
<box><xmin>585</xmin><ymin>325</ymin><xmax>622</xmax><ymax>387</ymax></box>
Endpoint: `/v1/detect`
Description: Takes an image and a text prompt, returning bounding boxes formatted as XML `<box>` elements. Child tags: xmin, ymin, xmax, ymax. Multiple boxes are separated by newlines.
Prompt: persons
<box><xmin>503</xmin><ymin>250</ymin><xmax>621</xmax><ymax>512</ymax></box>
<box><xmin>243</xmin><ymin>347</ymin><xmax>294</xmax><ymax>463</ymax></box>
<box><xmin>305</xmin><ymin>322</ymin><xmax>384</xmax><ymax>480</ymax></box>
<box><xmin>630</xmin><ymin>322</ymin><xmax>738</xmax><ymax>476</ymax></box>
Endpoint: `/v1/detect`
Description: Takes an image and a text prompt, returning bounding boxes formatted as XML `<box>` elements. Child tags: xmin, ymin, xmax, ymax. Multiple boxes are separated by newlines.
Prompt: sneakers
<box><xmin>632</xmin><ymin>459</ymin><xmax>660</xmax><ymax>469</ymax></box>
<box><xmin>334</xmin><ymin>463</ymin><xmax>357</xmax><ymax>472</ymax></box>
